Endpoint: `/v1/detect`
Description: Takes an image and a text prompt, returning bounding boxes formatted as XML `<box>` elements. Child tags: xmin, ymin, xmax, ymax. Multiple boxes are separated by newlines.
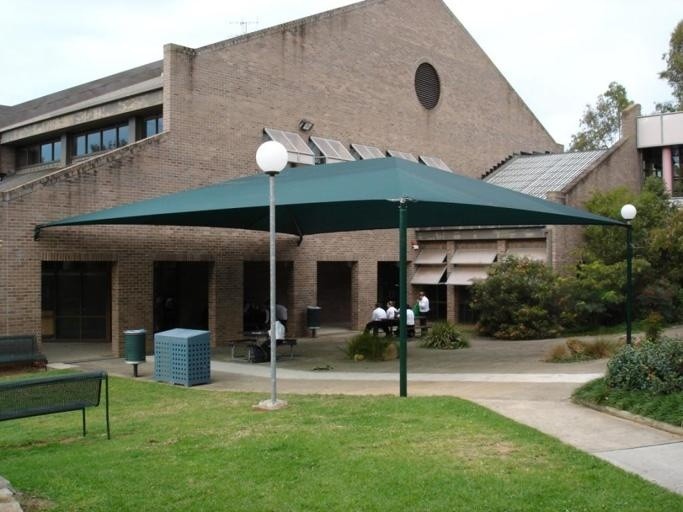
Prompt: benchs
<box><xmin>374</xmin><ymin>316</ymin><xmax>431</xmax><ymax>338</ymax></box>
<box><xmin>230</xmin><ymin>335</ymin><xmax>297</xmax><ymax>363</ymax></box>
<box><xmin>0</xmin><ymin>334</ymin><xmax>110</xmax><ymax>440</ymax></box>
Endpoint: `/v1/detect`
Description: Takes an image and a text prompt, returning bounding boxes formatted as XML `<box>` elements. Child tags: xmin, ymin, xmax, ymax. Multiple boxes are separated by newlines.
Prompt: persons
<box><xmin>243</xmin><ymin>300</ymin><xmax>288</xmax><ymax>332</ymax></box>
<box><xmin>261</xmin><ymin>318</ymin><xmax>287</xmax><ymax>362</ymax></box>
<box><xmin>362</xmin><ymin>291</ymin><xmax>431</xmax><ymax>336</ymax></box>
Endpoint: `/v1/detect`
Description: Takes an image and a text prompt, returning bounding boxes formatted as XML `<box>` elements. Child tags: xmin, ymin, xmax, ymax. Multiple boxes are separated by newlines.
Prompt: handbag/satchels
<box><xmin>413</xmin><ymin>302</ymin><xmax>420</xmax><ymax>316</ymax></box>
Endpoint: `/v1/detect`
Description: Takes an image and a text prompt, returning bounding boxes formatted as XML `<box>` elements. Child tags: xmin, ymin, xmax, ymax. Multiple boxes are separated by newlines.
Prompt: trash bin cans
<box><xmin>123</xmin><ymin>328</ymin><xmax>147</xmax><ymax>377</ymax></box>
<box><xmin>306</xmin><ymin>306</ymin><xmax>322</xmax><ymax>337</ymax></box>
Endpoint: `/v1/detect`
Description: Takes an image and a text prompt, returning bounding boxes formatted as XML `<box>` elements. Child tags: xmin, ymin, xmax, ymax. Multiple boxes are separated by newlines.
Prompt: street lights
<box><xmin>256</xmin><ymin>142</ymin><xmax>288</xmax><ymax>403</ymax></box>
<box><xmin>620</xmin><ymin>204</ymin><xmax>637</xmax><ymax>224</ymax></box>
